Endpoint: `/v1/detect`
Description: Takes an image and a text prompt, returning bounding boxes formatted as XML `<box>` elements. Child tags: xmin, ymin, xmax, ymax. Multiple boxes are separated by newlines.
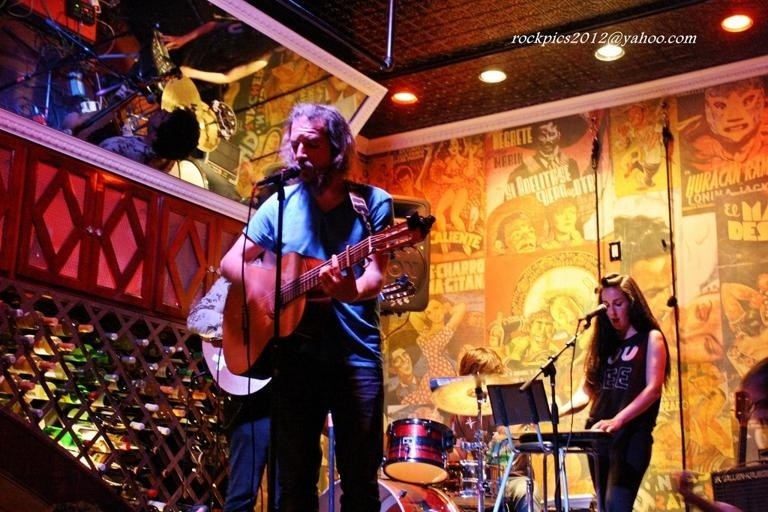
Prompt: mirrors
<box><xmin>0</xmin><ymin>0</ymin><xmax>389</xmax><ymax>223</ymax></box>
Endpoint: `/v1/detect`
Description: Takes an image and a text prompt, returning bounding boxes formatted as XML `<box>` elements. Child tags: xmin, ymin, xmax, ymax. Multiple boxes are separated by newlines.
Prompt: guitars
<box><xmin>202</xmin><ymin>276</ymin><xmax>418</xmax><ymax>396</ymax></box>
<box><xmin>735</xmin><ymin>389</ymin><xmax>753</xmax><ymax>466</ymax></box>
<box><xmin>222</xmin><ymin>214</ymin><xmax>435</xmax><ymax>377</ymax></box>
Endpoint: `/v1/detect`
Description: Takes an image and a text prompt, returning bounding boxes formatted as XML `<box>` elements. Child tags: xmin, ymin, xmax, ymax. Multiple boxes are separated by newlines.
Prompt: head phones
<box><xmin>312</xmin><ymin>104</ymin><xmax>356</xmax><ymax>173</ymax></box>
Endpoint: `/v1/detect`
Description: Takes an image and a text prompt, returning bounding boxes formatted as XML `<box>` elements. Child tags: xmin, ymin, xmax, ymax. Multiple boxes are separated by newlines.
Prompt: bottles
<box><xmin>0</xmin><ymin>300</ymin><xmax>231</xmax><ymax>512</ymax></box>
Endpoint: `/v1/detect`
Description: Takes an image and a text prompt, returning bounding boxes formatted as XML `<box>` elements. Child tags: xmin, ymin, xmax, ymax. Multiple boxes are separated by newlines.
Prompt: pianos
<box><xmin>519</xmin><ymin>432</ymin><xmax>615</xmax><ymax>446</ymax></box>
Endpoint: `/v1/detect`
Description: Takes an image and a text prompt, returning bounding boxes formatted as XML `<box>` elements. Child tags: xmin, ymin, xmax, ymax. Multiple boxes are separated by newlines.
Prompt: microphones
<box><xmin>579</xmin><ymin>303</ymin><xmax>608</xmax><ymax>320</ymax></box>
<box><xmin>255</xmin><ymin>165</ymin><xmax>303</xmax><ymax>188</ymax></box>
<box><xmin>500</xmin><ymin>315</ymin><xmax>524</xmax><ymax>330</ymax></box>
<box><xmin>131</xmin><ymin>74</ymin><xmax>157</xmax><ymax>103</ymax></box>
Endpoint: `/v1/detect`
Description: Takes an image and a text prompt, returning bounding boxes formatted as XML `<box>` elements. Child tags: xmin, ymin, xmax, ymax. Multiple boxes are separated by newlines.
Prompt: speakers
<box><xmin>381</xmin><ymin>194</ymin><xmax>431</xmax><ymax>312</ymax></box>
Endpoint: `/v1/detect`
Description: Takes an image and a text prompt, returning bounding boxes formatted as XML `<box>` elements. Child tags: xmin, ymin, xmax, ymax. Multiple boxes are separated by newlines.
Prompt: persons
<box><xmin>218</xmin><ymin>101</ymin><xmax>394</xmax><ymax>512</ymax></box>
<box><xmin>95</xmin><ymin>106</ymin><xmax>199</xmax><ymax>176</ymax></box>
<box><xmin>390</xmin><ymin>75</ymin><xmax>768</xmax><ymax>376</ymax></box>
<box><xmin>549</xmin><ymin>270</ymin><xmax>670</xmax><ymax>511</ymax></box>
<box><xmin>449</xmin><ymin>346</ymin><xmax>542</xmax><ymax>511</ymax></box>
<box><xmin>186</xmin><ymin>160</ymin><xmax>316</xmax><ymax>512</ymax></box>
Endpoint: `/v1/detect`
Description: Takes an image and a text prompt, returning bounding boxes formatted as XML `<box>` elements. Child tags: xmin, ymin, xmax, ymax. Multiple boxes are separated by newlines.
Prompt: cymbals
<box><xmin>161</xmin><ymin>76</ymin><xmax>203</xmax><ymax>124</ymax></box>
<box><xmin>432</xmin><ymin>375</ymin><xmax>515</xmax><ymax>417</ymax></box>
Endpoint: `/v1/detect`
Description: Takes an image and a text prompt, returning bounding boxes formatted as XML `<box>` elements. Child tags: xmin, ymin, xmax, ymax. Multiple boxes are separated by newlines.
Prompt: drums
<box><xmin>383</xmin><ymin>418</ymin><xmax>456</xmax><ymax>484</ymax></box>
<box><xmin>447</xmin><ymin>460</ymin><xmax>500</xmax><ymax>506</ymax></box>
<box><xmin>167</xmin><ymin>160</ymin><xmax>207</xmax><ymax>190</ymax></box>
<box><xmin>35</xmin><ymin>67</ymin><xmax>123</xmax><ymax>144</ymax></box>
<box><xmin>319</xmin><ymin>479</ymin><xmax>461</xmax><ymax>512</ymax></box>
